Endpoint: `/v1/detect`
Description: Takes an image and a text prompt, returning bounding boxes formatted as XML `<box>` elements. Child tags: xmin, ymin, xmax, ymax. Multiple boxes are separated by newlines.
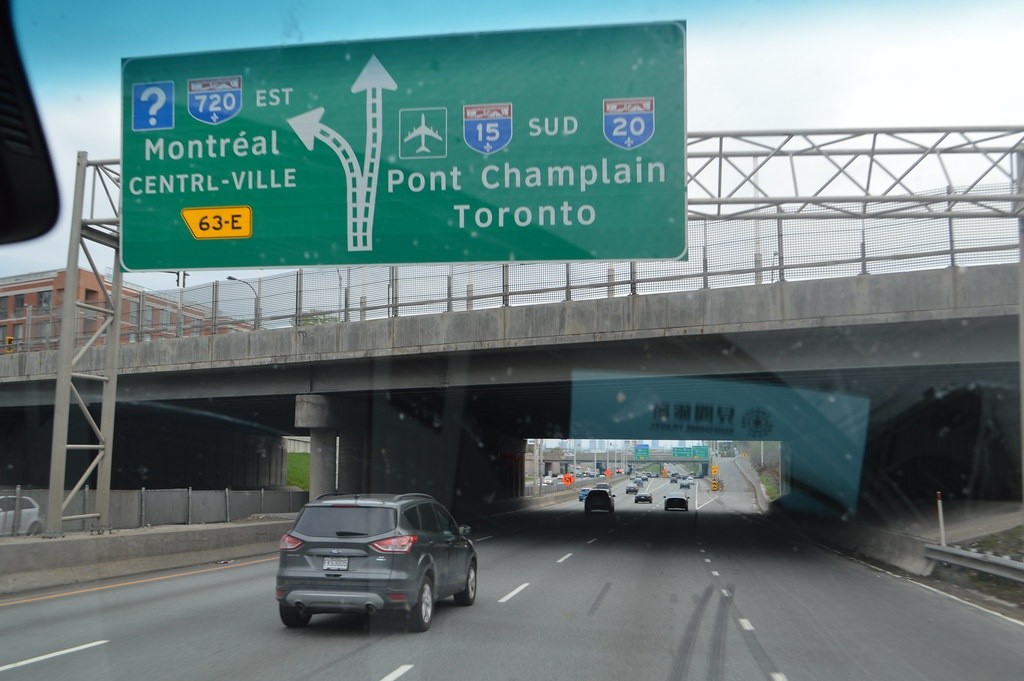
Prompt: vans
<box><xmin>579</xmin><ymin>487</ymin><xmax>591</xmax><ymax>501</ymax></box>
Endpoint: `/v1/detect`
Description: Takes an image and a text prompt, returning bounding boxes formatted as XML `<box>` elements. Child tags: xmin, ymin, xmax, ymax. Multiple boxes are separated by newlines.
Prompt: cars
<box><xmin>575</xmin><ymin>467</ymin><xmax>598</xmax><ymax>478</ymax></box>
<box><xmin>544</xmin><ymin>477</ymin><xmax>553</xmax><ymax>483</ymax></box>
<box><xmin>626</xmin><ymin>483</ymin><xmax>638</xmax><ymax>494</ymax></box>
<box><xmin>672</xmin><ymin>473</ymin><xmax>688</xmax><ymax>480</ymax></box>
<box><xmin>630</xmin><ymin>473</ymin><xmax>649</xmax><ymax>481</ymax></box>
<box><xmin>635</xmin><ymin>491</ymin><xmax>652</xmax><ymax>504</ymax></box>
<box><xmin>664</xmin><ymin>492</ymin><xmax>690</xmax><ymax>510</ymax></box>
<box><xmin>686</xmin><ymin>477</ymin><xmax>694</xmax><ymax>484</ymax></box>
<box><xmin>670</xmin><ymin>477</ymin><xmax>677</xmax><ymax>483</ymax></box>
<box><xmin>557</xmin><ymin>474</ymin><xmax>563</xmax><ymax>479</ymax></box>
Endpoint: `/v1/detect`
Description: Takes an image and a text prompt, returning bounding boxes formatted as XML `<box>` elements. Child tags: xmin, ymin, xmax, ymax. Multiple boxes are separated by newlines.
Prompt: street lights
<box><xmin>227</xmin><ymin>275</ymin><xmax>262</xmax><ymax>331</ymax></box>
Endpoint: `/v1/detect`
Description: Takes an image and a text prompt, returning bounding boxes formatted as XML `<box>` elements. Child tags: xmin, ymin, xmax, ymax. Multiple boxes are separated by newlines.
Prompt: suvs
<box><xmin>275</xmin><ymin>493</ymin><xmax>477</xmax><ymax>632</ymax></box>
<box><xmin>586</xmin><ymin>489</ymin><xmax>615</xmax><ymax>513</ymax></box>
<box><xmin>0</xmin><ymin>495</ymin><xmax>42</xmax><ymax>537</ymax></box>
<box><xmin>680</xmin><ymin>480</ymin><xmax>691</xmax><ymax>489</ymax></box>
<box><xmin>633</xmin><ymin>478</ymin><xmax>643</xmax><ymax>487</ymax></box>
<box><xmin>594</xmin><ymin>482</ymin><xmax>611</xmax><ymax>490</ymax></box>
